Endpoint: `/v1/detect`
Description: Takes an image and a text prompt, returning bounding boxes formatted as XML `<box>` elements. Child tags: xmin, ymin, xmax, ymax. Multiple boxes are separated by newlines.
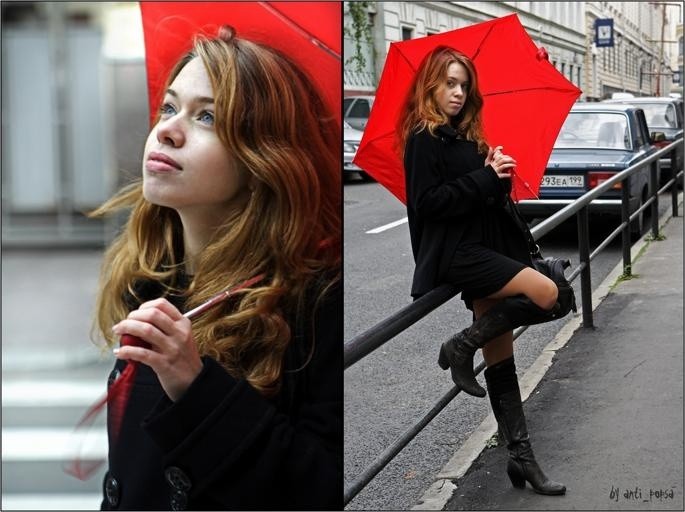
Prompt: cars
<box><xmin>343</xmin><ymin>121</ymin><xmax>364</xmax><ymax>184</ymax></box>
<box><xmin>518</xmin><ymin>95</ymin><xmax>685</xmax><ymax>240</ymax></box>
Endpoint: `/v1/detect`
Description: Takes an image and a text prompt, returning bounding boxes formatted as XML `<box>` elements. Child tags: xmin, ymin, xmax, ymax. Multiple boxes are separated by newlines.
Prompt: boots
<box><xmin>437</xmin><ymin>299</ymin><xmax>531</xmax><ymax>398</ymax></box>
<box><xmin>482</xmin><ymin>355</ymin><xmax>567</xmax><ymax>497</ymax></box>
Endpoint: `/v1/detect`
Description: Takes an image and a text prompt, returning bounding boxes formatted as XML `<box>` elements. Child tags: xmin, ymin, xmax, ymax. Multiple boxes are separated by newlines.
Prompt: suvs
<box><xmin>344</xmin><ymin>96</ymin><xmax>376</xmax><ymax>132</ymax></box>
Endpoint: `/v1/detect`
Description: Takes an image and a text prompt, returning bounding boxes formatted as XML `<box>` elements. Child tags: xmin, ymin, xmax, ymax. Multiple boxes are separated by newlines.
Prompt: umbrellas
<box><xmin>121</xmin><ymin>1</ymin><xmax>343</xmax><ymax>363</ymax></box>
<box><xmin>352</xmin><ymin>12</ymin><xmax>583</xmax><ymax>209</ymax></box>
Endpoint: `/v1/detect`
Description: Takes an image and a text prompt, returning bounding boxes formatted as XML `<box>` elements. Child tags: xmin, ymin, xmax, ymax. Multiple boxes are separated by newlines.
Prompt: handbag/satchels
<box><xmin>510</xmin><ymin>195</ymin><xmax>575</xmax><ymax>330</ymax></box>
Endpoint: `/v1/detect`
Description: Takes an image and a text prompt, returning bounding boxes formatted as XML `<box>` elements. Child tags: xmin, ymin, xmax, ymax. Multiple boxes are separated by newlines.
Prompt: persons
<box><xmin>395</xmin><ymin>40</ymin><xmax>576</xmax><ymax>498</ymax></box>
<box><xmin>81</xmin><ymin>19</ymin><xmax>344</xmax><ymax>512</ymax></box>
